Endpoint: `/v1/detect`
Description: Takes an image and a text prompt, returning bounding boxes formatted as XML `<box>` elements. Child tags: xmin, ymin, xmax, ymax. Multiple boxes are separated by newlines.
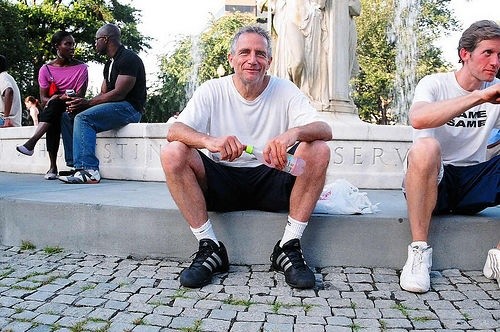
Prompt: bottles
<box><xmin>245</xmin><ymin>144</ymin><xmax>306</xmax><ymax>177</ymax></box>
<box><xmin>66</xmin><ymin>89</ymin><xmax>75</xmax><ymax>101</ymax></box>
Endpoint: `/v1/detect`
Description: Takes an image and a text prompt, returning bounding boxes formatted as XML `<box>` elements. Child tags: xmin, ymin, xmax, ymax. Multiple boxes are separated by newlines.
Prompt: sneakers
<box><xmin>58</xmin><ymin>169</ymin><xmax>101</xmax><ymax>183</ymax></box>
<box><xmin>180</xmin><ymin>238</ymin><xmax>230</xmax><ymax>288</ymax></box>
<box><xmin>400</xmin><ymin>241</ymin><xmax>432</xmax><ymax>294</ymax></box>
<box><xmin>483</xmin><ymin>248</ymin><xmax>500</xmax><ymax>289</ymax></box>
<box><xmin>270</xmin><ymin>238</ymin><xmax>315</xmax><ymax>288</ymax></box>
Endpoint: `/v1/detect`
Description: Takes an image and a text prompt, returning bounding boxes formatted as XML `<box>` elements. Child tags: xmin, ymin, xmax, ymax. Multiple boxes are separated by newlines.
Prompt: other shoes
<box><xmin>45</xmin><ymin>167</ymin><xmax>57</xmax><ymax>180</ymax></box>
<box><xmin>17</xmin><ymin>145</ymin><xmax>35</xmax><ymax>156</ymax></box>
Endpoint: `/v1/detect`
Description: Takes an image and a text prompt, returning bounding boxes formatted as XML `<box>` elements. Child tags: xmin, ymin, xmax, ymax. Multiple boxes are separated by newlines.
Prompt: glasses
<box><xmin>95</xmin><ymin>37</ymin><xmax>106</xmax><ymax>42</ymax></box>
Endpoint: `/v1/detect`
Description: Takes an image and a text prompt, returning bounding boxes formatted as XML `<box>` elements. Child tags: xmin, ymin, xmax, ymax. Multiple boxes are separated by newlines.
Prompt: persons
<box><xmin>24</xmin><ymin>96</ymin><xmax>43</xmax><ymax>126</ymax></box>
<box><xmin>400</xmin><ymin>20</ymin><xmax>500</xmax><ymax>293</ymax></box>
<box><xmin>258</xmin><ymin>0</ymin><xmax>361</xmax><ymax>113</ymax></box>
<box><xmin>159</xmin><ymin>26</ymin><xmax>333</xmax><ymax>290</ymax></box>
<box><xmin>0</xmin><ymin>55</ymin><xmax>22</xmax><ymax>128</ymax></box>
<box><xmin>59</xmin><ymin>24</ymin><xmax>147</xmax><ymax>183</ymax></box>
<box><xmin>17</xmin><ymin>31</ymin><xmax>88</xmax><ymax>180</ymax></box>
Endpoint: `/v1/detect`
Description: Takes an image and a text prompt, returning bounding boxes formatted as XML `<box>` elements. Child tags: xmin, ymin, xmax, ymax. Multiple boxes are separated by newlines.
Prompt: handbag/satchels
<box><xmin>313</xmin><ymin>179</ymin><xmax>365</xmax><ymax>214</ymax></box>
<box><xmin>45</xmin><ymin>65</ymin><xmax>60</xmax><ymax>98</ymax></box>
